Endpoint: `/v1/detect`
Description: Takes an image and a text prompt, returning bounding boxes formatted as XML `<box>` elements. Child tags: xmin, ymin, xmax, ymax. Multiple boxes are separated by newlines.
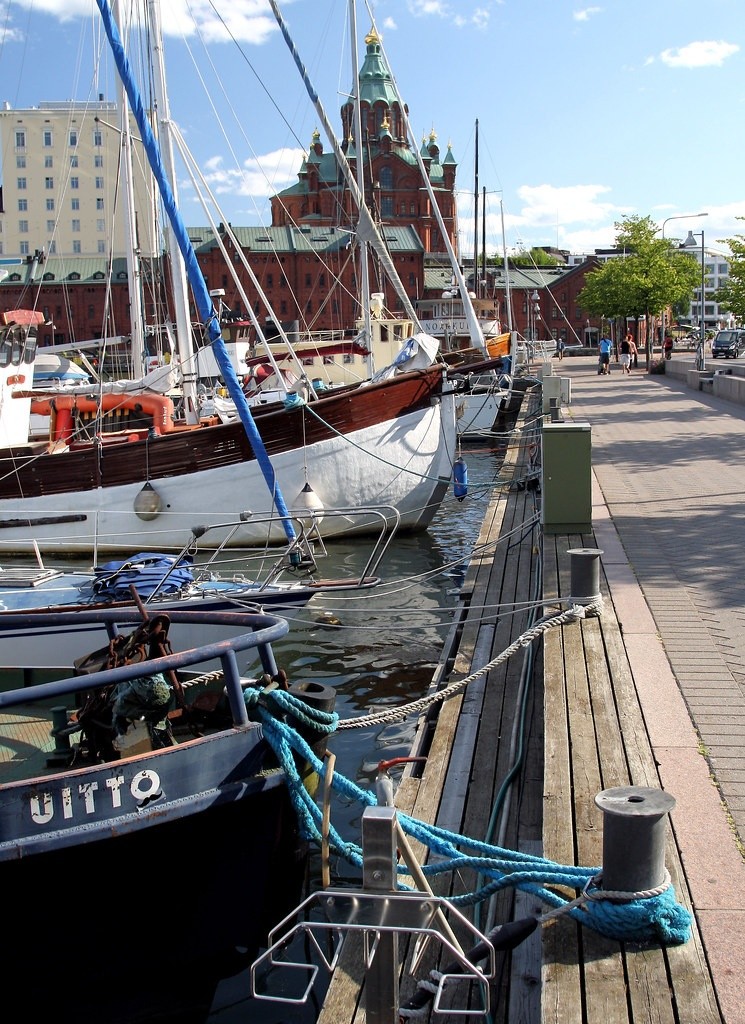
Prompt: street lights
<box><xmin>662</xmin><ymin>212</ymin><xmax>708</xmax><ymax>238</ymax></box>
<box><xmin>683</xmin><ymin>230</ymin><xmax>707</xmax><ymax>371</ymax></box>
<box><xmin>711</xmin><ymin>254</ymin><xmax>724</xmax><ymax>257</ymax></box>
<box><xmin>525</xmin><ymin>289</ymin><xmax>542</xmax><ymax>361</ymax></box>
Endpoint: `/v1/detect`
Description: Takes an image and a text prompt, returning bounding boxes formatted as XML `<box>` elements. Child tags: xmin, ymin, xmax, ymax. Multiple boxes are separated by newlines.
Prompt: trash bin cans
<box><xmin>540</xmin><ymin>419</ymin><xmax>594</xmax><ymax>534</ymax></box>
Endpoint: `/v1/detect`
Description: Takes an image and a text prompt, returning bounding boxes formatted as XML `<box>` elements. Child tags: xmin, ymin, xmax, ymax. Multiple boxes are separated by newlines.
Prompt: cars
<box><xmin>686</xmin><ymin>327</ymin><xmax>700</xmax><ymax>339</ymax></box>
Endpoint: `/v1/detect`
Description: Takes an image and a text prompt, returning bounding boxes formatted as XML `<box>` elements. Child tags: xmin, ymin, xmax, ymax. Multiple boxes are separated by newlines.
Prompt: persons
<box><xmin>627</xmin><ymin>334</ymin><xmax>638</xmax><ymax>373</ymax></box>
<box><xmin>618</xmin><ymin>335</ymin><xmax>634</xmax><ymax>374</ymax></box>
<box><xmin>597</xmin><ymin>334</ymin><xmax>612</xmax><ymax>375</ymax></box>
<box><xmin>663</xmin><ymin>334</ymin><xmax>673</xmax><ymax>360</ymax></box>
<box><xmin>556</xmin><ymin>338</ymin><xmax>565</xmax><ymax>360</ymax></box>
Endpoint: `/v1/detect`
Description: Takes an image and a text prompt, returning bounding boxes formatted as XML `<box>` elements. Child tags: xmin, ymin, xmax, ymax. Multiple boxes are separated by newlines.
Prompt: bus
<box><xmin>671</xmin><ymin>325</ymin><xmax>693</xmax><ymax>337</ymax></box>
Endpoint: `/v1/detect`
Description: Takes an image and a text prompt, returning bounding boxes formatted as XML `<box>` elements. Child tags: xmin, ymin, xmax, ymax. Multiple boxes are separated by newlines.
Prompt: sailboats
<box><xmin>1</xmin><ymin>4</ymin><xmax>532</xmax><ymax>558</ymax></box>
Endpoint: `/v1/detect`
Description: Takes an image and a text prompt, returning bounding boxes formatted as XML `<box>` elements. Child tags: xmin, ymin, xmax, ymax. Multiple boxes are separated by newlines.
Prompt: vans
<box><xmin>711</xmin><ymin>330</ymin><xmax>745</xmax><ymax>359</ymax></box>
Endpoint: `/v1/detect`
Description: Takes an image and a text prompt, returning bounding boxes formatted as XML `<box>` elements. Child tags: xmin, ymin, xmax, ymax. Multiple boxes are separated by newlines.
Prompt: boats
<box><xmin>0</xmin><ymin>4</ymin><xmax>340</xmax><ymax>925</ymax></box>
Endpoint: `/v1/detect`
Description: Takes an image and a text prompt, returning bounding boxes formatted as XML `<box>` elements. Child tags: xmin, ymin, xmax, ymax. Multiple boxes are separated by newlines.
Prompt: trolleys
<box><xmin>597</xmin><ymin>355</ymin><xmax>611</xmax><ymax>375</ymax></box>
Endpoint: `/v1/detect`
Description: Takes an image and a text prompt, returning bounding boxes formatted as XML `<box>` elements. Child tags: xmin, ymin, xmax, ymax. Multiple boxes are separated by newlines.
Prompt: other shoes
<box><xmin>603</xmin><ymin>368</ymin><xmax>607</xmax><ymax>375</ymax></box>
<box><xmin>626</xmin><ymin>368</ymin><xmax>629</xmax><ymax>373</ymax></box>
<box><xmin>607</xmin><ymin>370</ymin><xmax>610</xmax><ymax>375</ymax></box>
